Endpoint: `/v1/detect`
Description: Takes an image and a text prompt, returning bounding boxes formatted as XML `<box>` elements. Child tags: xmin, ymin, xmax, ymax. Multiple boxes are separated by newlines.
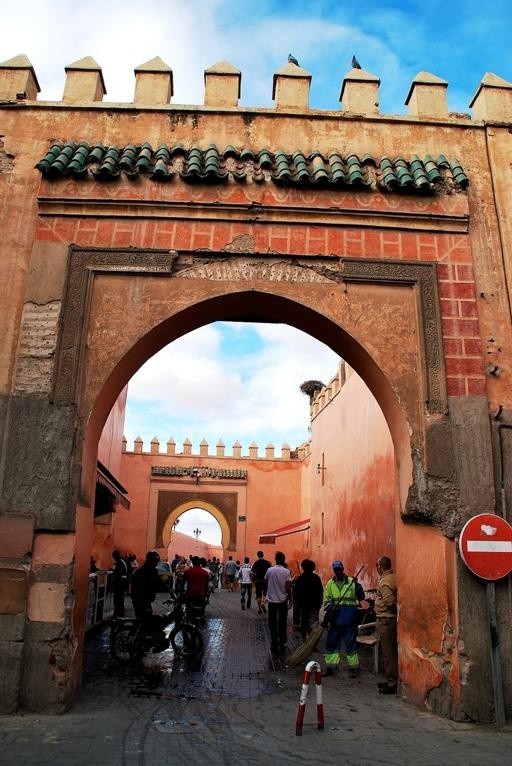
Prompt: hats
<box><xmin>332</xmin><ymin>559</ymin><xmax>344</xmax><ymax>571</ymax></box>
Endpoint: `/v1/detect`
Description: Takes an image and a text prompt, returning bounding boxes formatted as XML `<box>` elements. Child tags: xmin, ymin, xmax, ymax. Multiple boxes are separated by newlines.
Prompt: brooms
<box><xmin>283</xmin><ymin>565</ymin><xmax>366</xmax><ymax>668</ymax></box>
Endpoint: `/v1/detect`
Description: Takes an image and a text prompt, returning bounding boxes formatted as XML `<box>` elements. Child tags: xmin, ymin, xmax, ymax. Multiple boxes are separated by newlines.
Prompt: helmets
<box><xmin>146</xmin><ymin>550</ymin><xmax>161</xmax><ymax>562</ymax></box>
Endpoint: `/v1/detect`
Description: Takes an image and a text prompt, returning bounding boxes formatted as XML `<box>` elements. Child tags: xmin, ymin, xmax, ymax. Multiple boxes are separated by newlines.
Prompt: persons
<box><xmin>293</xmin><ymin>558</ymin><xmax>325</xmax><ymax>644</ymax></box>
<box><xmin>316</xmin><ymin>560</ymin><xmax>366</xmax><ymax>678</ymax></box>
<box><xmin>108</xmin><ymin>549</ymin><xmax>295</xmax><ymax>617</ymax></box>
<box><xmin>372</xmin><ymin>555</ymin><xmax>397</xmax><ymax>696</ymax></box>
<box><xmin>264</xmin><ymin>551</ymin><xmax>292</xmax><ymax>646</ymax></box>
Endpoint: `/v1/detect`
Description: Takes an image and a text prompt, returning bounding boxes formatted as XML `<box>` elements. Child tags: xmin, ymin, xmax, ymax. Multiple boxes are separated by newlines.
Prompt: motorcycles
<box><xmin>110</xmin><ymin>592</ymin><xmax>204</xmax><ymax>665</ymax></box>
<box><xmin>211</xmin><ymin>565</ymin><xmax>220</xmax><ymax>594</ymax></box>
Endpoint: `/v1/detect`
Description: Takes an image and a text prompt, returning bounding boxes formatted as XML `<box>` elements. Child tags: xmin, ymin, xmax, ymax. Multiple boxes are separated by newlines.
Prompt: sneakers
<box><xmin>240</xmin><ymin>602</ymin><xmax>267</xmax><ymax>615</ymax></box>
<box><xmin>377</xmin><ymin>680</ymin><xmax>397</xmax><ymax>695</ymax></box>
<box><xmin>322</xmin><ymin>667</ymin><xmax>360</xmax><ymax>679</ymax></box>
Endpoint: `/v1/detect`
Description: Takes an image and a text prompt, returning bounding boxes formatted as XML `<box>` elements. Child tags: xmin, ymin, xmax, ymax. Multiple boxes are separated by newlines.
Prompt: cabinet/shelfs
<box><xmin>84</xmin><ymin>570</ymin><xmax>114</xmax><ymax>632</ymax></box>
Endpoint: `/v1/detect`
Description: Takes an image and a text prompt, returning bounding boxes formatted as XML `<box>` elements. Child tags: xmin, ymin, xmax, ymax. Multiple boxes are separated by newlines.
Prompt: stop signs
<box><xmin>459</xmin><ymin>514</ymin><xmax>512</xmax><ymax>581</ymax></box>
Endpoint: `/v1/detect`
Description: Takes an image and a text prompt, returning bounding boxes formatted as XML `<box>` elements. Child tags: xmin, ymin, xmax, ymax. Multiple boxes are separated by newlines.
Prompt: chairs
<box><xmin>357</xmin><ymin>622</ymin><xmax>382</xmax><ymax>676</ymax></box>
